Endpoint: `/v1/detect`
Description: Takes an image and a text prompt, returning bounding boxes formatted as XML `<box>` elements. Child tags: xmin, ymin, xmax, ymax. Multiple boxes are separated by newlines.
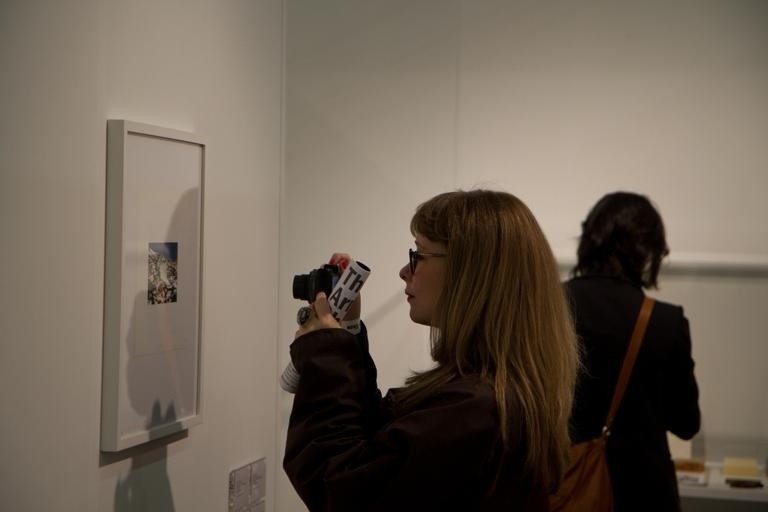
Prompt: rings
<box><xmin>297</xmin><ymin>306</ymin><xmax>314</xmax><ymax>328</ymax></box>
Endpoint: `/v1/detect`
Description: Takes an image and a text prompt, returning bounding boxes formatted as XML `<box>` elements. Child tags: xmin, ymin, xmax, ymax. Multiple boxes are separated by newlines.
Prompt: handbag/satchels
<box><xmin>546</xmin><ymin>435</ymin><xmax>611</xmax><ymax>511</ymax></box>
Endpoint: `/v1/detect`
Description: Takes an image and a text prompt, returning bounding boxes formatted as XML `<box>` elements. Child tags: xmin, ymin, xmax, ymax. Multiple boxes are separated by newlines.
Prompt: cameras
<box><xmin>293</xmin><ymin>264</ymin><xmax>340</xmax><ymax>307</ymax></box>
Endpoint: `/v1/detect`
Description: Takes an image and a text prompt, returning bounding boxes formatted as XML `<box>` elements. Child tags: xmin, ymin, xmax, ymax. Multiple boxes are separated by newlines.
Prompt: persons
<box><xmin>556</xmin><ymin>193</ymin><xmax>702</xmax><ymax>512</ymax></box>
<box><xmin>282</xmin><ymin>189</ymin><xmax>579</xmax><ymax>512</ymax></box>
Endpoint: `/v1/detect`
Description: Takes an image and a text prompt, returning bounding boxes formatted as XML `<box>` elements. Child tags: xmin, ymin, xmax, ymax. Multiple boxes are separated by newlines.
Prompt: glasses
<box><xmin>408</xmin><ymin>247</ymin><xmax>445</xmax><ymax>275</ymax></box>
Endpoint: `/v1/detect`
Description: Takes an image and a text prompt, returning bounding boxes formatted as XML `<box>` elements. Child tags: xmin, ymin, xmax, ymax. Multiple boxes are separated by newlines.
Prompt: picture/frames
<box><xmin>100</xmin><ymin>118</ymin><xmax>209</xmax><ymax>451</ymax></box>
